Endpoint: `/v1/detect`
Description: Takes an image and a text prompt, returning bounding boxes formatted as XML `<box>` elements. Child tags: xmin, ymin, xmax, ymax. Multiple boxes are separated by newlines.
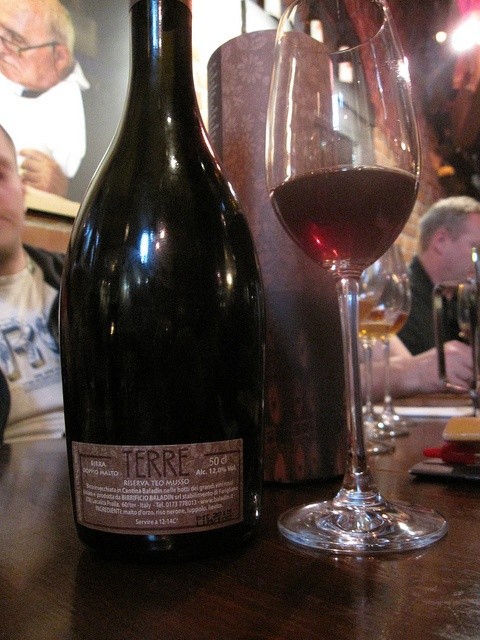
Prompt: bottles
<box><xmin>58</xmin><ymin>0</ymin><xmax>266</xmax><ymax>564</ymax></box>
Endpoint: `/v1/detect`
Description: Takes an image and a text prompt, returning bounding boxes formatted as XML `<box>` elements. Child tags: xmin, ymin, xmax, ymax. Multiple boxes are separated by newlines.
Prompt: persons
<box><xmin>0</xmin><ymin>123</ymin><xmax>66</xmax><ymax>448</ymax></box>
<box><xmin>345</xmin><ymin>332</ymin><xmax>473</xmax><ymax>398</ymax></box>
<box><xmin>0</xmin><ymin>1</ymin><xmax>106</xmax><ymax>201</ymax></box>
<box><xmin>391</xmin><ymin>194</ymin><xmax>480</xmax><ymax>358</ymax></box>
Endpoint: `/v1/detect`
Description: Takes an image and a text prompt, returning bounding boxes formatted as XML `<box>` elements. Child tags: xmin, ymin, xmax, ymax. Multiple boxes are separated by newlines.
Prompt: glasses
<box><xmin>0</xmin><ymin>37</ymin><xmax>56</xmax><ymax>57</ymax></box>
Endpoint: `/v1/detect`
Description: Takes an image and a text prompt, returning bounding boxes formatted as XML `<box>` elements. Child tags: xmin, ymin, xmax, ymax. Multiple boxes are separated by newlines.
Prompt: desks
<box><xmin>1</xmin><ymin>394</ymin><xmax>480</xmax><ymax>638</ymax></box>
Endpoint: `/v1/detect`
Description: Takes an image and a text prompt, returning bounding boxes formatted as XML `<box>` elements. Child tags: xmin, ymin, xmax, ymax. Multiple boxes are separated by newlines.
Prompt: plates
<box><xmin>409</xmin><ymin>455</ymin><xmax>479</xmax><ymax>482</ymax></box>
<box><xmin>360</xmin><ymin>406</ymin><xmax>476</xmax><ymax>418</ymax></box>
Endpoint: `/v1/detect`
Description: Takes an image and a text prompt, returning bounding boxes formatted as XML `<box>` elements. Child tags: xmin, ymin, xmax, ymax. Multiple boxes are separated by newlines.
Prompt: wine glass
<box><xmin>363</xmin><ymin>249</ymin><xmax>409</xmax><ymax>439</ymax></box>
<box><xmin>262</xmin><ymin>0</ymin><xmax>448</xmax><ymax>556</ymax></box>
<box><xmin>456</xmin><ymin>282</ymin><xmax>480</xmax><ymax>406</ymax></box>
<box><xmin>342</xmin><ymin>245</ymin><xmax>388</xmax><ymax>454</ymax></box>
